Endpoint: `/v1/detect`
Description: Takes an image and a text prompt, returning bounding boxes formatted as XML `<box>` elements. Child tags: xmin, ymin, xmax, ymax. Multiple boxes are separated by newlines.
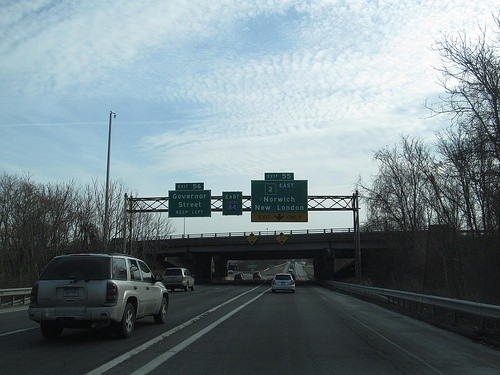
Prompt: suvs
<box><xmin>162</xmin><ymin>267</ymin><xmax>195</xmax><ymax>292</ymax></box>
<box><xmin>28</xmin><ymin>253</ymin><xmax>170</xmax><ymax>338</ymax></box>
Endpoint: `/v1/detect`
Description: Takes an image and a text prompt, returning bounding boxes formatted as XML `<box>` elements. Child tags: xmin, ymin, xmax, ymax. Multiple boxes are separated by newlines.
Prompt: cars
<box><xmin>234</xmin><ymin>273</ymin><xmax>243</xmax><ymax>280</ymax></box>
<box><xmin>271</xmin><ymin>273</ymin><xmax>295</xmax><ymax>294</ymax></box>
<box><xmin>253</xmin><ymin>271</ymin><xmax>261</xmax><ymax>280</ymax></box>
<box><xmin>287</xmin><ymin>262</ymin><xmax>296</xmax><ymax>284</ymax></box>
<box><xmin>228</xmin><ymin>270</ymin><xmax>233</xmax><ymax>274</ymax></box>
